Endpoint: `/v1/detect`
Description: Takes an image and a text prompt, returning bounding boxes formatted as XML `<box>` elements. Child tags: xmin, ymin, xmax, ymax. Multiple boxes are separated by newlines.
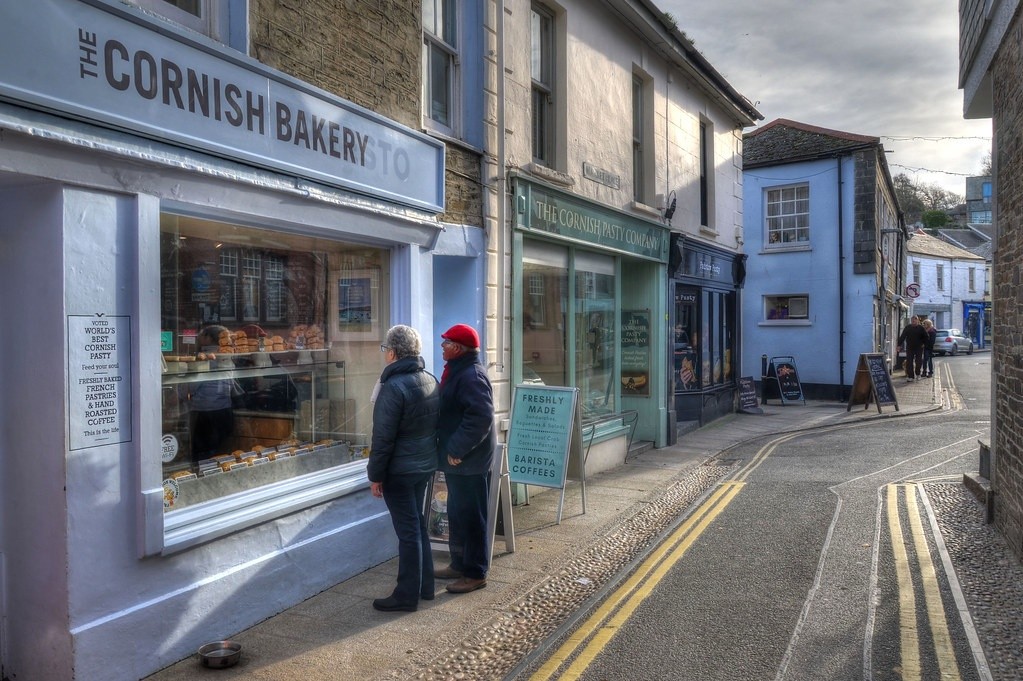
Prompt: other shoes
<box><xmin>446</xmin><ymin>577</ymin><xmax>488</xmax><ymax>593</ymax></box>
<box><xmin>916</xmin><ymin>375</ymin><xmax>920</xmax><ymax>380</ymax></box>
<box><xmin>927</xmin><ymin>372</ymin><xmax>933</xmax><ymax>378</ymax></box>
<box><xmin>921</xmin><ymin>371</ymin><xmax>927</xmax><ymax>377</ymax></box>
<box><xmin>422</xmin><ymin>592</ymin><xmax>434</xmax><ymax>601</ymax></box>
<box><xmin>373</xmin><ymin>593</ymin><xmax>417</xmax><ymax>611</ymax></box>
<box><xmin>435</xmin><ymin>567</ymin><xmax>463</xmax><ymax>579</ymax></box>
<box><xmin>907</xmin><ymin>378</ymin><xmax>913</xmax><ymax>382</ymax></box>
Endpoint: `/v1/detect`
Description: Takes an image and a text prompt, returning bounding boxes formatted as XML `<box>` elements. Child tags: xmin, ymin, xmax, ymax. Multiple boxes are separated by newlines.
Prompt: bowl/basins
<box><xmin>198</xmin><ymin>640</ymin><xmax>242</xmax><ymax>668</ymax></box>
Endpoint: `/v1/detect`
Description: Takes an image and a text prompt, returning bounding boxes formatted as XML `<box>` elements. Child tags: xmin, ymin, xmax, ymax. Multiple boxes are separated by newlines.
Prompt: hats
<box><xmin>441</xmin><ymin>324</ymin><xmax>479</xmax><ymax>348</ymax></box>
<box><xmin>242</xmin><ymin>324</ymin><xmax>267</xmax><ymax>338</ymax></box>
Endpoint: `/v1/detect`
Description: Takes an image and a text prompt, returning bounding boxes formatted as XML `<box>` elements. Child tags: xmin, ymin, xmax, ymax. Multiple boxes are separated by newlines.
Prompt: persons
<box><xmin>184</xmin><ymin>323</ymin><xmax>239</xmax><ymax>461</ymax></box>
<box><xmin>921</xmin><ymin>319</ymin><xmax>937</xmax><ymax>378</ymax></box>
<box><xmin>367</xmin><ymin>325</ymin><xmax>442</xmax><ymax>612</ymax></box>
<box><xmin>193</xmin><ymin>324</ymin><xmax>298</xmax><ymax>449</ymax></box>
<box><xmin>671</xmin><ymin>322</ymin><xmax>699</xmax><ymax>390</ymax></box>
<box><xmin>896</xmin><ymin>315</ymin><xmax>932</xmax><ymax>382</ymax></box>
<box><xmin>434</xmin><ymin>325</ymin><xmax>496</xmax><ymax>593</ymax></box>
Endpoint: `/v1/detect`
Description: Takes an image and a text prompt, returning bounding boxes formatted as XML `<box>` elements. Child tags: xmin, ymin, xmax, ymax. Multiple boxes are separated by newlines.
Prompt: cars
<box><xmin>523</xmin><ymin>364</ymin><xmax>546</xmax><ymax>386</ymax></box>
<box><xmin>933</xmin><ymin>329</ymin><xmax>973</xmax><ymax>356</ymax></box>
<box><xmin>675</xmin><ymin>328</ymin><xmax>689</xmax><ymax>351</ymax></box>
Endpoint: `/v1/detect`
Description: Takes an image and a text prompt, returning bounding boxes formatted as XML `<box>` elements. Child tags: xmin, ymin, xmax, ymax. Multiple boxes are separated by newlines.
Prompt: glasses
<box><xmin>381</xmin><ymin>344</ymin><xmax>388</xmax><ymax>352</ymax></box>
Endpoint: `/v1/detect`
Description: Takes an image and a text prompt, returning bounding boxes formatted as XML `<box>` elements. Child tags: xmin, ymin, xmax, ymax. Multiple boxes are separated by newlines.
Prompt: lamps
<box><xmin>663</xmin><ymin>189</ymin><xmax>678</xmax><ymax>225</ymax></box>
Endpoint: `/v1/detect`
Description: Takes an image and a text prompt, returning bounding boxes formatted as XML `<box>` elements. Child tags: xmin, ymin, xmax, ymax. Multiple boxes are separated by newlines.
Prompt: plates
<box><xmin>431</xmin><ymin>499</ymin><xmax>447</xmax><ymax>513</ymax></box>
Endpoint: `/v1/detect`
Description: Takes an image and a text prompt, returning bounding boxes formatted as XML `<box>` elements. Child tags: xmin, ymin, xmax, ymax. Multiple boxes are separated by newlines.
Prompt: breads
<box><xmin>170</xmin><ymin>439</ymin><xmax>335</xmax><ymax>480</ymax></box>
<box><xmin>217</xmin><ymin>323</ymin><xmax>325</xmax><ymax>354</ymax></box>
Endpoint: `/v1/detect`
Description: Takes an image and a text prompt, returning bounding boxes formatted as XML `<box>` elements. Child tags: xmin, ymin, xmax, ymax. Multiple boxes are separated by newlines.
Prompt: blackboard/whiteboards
<box><xmin>848</xmin><ymin>353</ymin><xmax>898</xmax><ymax>405</ymax></box>
<box><xmin>736</xmin><ymin>375</ymin><xmax>759</xmax><ymax>410</ymax></box>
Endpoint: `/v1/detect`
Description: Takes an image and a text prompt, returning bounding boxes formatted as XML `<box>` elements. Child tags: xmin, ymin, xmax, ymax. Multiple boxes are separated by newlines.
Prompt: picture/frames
<box><xmin>327</xmin><ymin>264</ymin><xmax>384</xmax><ymax>343</ymax></box>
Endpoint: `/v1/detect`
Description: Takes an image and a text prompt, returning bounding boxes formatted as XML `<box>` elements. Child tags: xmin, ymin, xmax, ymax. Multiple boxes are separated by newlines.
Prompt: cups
<box><xmin>435</xmin><ymin>491</ymin><xmax>448</xmax><ymax>507</ymax></box>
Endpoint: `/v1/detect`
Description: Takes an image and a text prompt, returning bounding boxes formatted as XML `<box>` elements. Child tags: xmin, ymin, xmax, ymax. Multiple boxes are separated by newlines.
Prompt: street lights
<box><xmin>560</xmin><ymin>293</ymin><xmax>567</xmax><ymax>386</ymax></box>
